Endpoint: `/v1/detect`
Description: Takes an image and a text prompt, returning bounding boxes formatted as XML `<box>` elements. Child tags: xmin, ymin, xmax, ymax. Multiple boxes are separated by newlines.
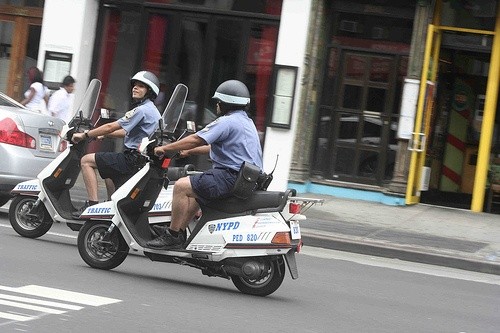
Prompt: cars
<box><xmin>317</xmin><ymin>115</ymin><xmax>398</xmax><ymax>175</ymax></box>
<box><xmin>0</xmin><ymin>90</ymin><xmax>68</xmax><ymax>208</ymax></box>
<box><xmin>181</xmin><ymin>99</ymin><xmax>216</xmax><ymax>127</ymax></box>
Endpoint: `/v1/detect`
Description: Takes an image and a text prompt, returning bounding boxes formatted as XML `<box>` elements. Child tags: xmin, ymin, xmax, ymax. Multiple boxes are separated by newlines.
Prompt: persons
<box><xmin>146</xmin><ymin>80</ymin><xmax>263</xmax><ymax>249</ymax></box>
<box><xmin>71</xmin><ymin>70</ymin><xmax>162</xmax><ymax>216</ymax></box>
<box><xmin>48</xmin><ymin>76</ymin><xmax>75</xmax><ymax>119</ymax></box>
<box><xmin>20</xmin><ymin>67</ymin><xmax>47</xmax><ymax>114</ymax></box>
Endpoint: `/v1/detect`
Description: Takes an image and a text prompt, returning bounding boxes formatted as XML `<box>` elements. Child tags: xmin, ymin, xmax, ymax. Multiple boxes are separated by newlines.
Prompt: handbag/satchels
<box><xmin>231</xmin><ymin>161</ymin><xmax>261</xmax><ymax>201</ymax></box>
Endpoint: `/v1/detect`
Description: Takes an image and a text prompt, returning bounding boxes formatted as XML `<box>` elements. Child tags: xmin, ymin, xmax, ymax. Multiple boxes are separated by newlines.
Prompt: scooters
<box><xmin>78</xmin><ymin>84</ymin><xmax>325</xmax><ymax>297</ymax></box>
<box><xmin>8</xmin><ymin>79</ymin><xmax>203</xmax><ymax>241</ymax></box>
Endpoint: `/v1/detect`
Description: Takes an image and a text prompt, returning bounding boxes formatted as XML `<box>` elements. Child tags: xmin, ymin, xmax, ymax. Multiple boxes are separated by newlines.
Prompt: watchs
<box><xmin>84</xmin><ymin>129</ymin><xmax>89</xmax><ymax>138</ymax></box>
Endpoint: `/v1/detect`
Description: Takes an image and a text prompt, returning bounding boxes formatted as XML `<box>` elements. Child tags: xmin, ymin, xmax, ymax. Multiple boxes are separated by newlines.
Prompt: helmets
<box><xmin>211</xmin><ymin>79</ymin><xmax>250</xmax><ymax>105</ymax></box>
<box><xmin>131</xmin><ymin>70</ymin><xmax>160</xmax><ymax>97</ymax></box>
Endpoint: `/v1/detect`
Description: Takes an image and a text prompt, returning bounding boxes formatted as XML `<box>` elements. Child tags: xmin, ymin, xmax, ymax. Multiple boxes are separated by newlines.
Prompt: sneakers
<box><xmin>146</xmin><ymin>230</ymin><xmax>184</xmax><ymax>248</ymax></box>
<box><xmin>71</xmin><ymin>200</ymin><xmax>98</xmax><ymax>218</ymax></box>
<box><xmin>152</xmin><ymin>225</ymin><xmax>187</xmax><ymax>243</ymax></box>
